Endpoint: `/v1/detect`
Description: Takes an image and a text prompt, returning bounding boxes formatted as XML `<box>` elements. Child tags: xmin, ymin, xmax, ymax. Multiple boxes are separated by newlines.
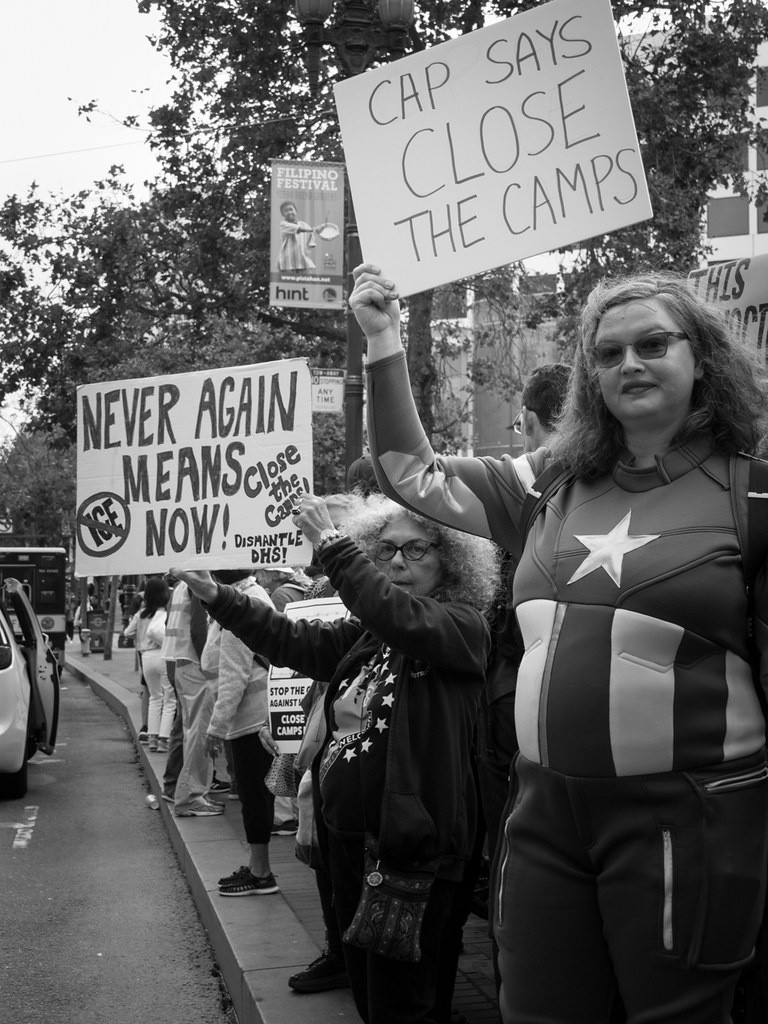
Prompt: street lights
<box><xmin>290</xmin><ymin>0</ymin><xmax>418</xmax><ymax>490</ymax></box>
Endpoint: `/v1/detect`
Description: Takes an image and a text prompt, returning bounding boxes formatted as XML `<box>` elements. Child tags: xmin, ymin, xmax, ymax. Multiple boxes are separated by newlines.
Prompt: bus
<box><xmin>0</xmin><ymin>546</ymin><xmax>67</xmax><ymax>678</ymax></box>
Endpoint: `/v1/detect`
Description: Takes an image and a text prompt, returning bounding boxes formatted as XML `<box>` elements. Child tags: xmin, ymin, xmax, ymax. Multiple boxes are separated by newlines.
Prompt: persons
<box><xmin>125</xmin><ymin>265</ymin><xmax>768</xmax><ymax>1024</ymax></box>
<box><xmin>278</xmin><ymin>201</ymin><xmax>325</xmax><ymax>274</ymax></box>
<box><xmin>66</xmin><ymin>583</ymin><xmax>103</xmax><ymax>645</ymax></box>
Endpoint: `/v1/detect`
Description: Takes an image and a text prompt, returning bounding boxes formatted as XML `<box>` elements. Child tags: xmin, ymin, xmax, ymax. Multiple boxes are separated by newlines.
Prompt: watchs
<box><xmin>313</xmin><ymin>528</ymin><xmax>340</xmax><ymax>551</ymax></box>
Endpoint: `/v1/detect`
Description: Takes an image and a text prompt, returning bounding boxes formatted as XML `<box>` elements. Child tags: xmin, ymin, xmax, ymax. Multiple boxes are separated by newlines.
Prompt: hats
<box><xmin>346</xmin><ymin>455</ymin><xmax>381</xmax><ymax>498</ymax></box>
<box><xmin>264</xmin><ymin>568</ymin><xmax>295</xmax><ymax>574</ymax></box>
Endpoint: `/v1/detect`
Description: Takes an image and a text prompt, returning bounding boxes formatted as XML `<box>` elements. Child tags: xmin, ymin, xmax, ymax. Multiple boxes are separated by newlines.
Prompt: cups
<box><xmin>144</xmin><ymin>793</ymin><xmax>159</xmax><ymax>810</ymax></box>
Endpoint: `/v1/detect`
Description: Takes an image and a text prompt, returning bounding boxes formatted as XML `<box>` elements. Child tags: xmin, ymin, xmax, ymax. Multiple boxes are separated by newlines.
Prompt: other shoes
<box><xmin>148</xmin><ymin>738</ymin><xmax>170</xmax><ymax>752</ymax></box>
<box><xmin>162</xmin><ymin>790</ymin><xmax>175</xmax><ymax>803</ymax></box>
<box><xmin>138</xmin><ymin>725</ymin><xmax>149</xmax><ymax>744</ymax></box>
<box><xmin>269</xmin><ymin>820</ymin><xmax>298</xmax><ymax>835</ymax></box>
<box><xmin>227</xmin><ymin>782</ymin><xmax>239</xmax><ymax>799</ymax></box>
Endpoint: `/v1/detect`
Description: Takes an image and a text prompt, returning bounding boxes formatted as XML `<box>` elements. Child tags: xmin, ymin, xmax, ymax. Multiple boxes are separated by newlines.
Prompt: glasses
<box><xmin>584</xmin><ymin>332</ymin><xmax>691</xmax><ymax>368</ymax></box>
<box><xmin>375</xmin><ymin>539</ymin><xmax>439</xmax><ymax>561</ymax></box>
<box><xmin>511</xmin><ymin>411</ymin><xmax>523</xmax><ymax>435</ymax></box>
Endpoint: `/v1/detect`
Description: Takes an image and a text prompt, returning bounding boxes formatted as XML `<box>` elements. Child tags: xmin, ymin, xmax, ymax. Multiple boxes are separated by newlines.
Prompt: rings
<box><xmin>291</xmin><ymin>506</ymin><xmax>301</xmax><ymax>515</ymax></box>
<box><xmin>299</xmin><ymin>498</ymin><xmax>304</xmax><ymax>504</ymax></box>
<box><xmin>214</xmin><ymin>748</ymin><xmax>218</xmax><ymax>751</ymax></box>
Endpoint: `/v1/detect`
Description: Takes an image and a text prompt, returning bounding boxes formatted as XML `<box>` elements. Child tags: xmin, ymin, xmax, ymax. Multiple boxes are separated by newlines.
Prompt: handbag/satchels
<box><xmin>341</xmin><ymin>827</ymin><xmax>436</xmax><ymax>963</ymax></box>
<box><xmin>264</xmin><ymin>752</ymin><xmax>302</xmax><ymax>797</ymax></box>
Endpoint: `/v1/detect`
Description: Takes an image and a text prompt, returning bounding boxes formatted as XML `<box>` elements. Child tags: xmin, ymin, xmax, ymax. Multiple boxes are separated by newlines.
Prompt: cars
<box><xmin>0</xmin><ymin>575</ymin><xmax>60</xmax><ymax>798</ymax></box>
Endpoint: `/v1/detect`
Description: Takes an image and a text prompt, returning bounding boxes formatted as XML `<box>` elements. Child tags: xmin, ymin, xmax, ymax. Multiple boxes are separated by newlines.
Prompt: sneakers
<box><xmin>289</xmin><ymin>950</ymin><xmax>351</xmax><ymax>993</ymax></box>
<box><xmin>175</xmin><ymin>795</ymin><xmax>225</xmax><ymax>816</ymax></box>
<box><xmin>218</xmin><ymin>866</ymin><xmax>280</xmax><ymax>897</ymax></box>
<box><xmin>208</xmin><ymin>770</ymin><xmax>230</xmax><ymax>793</ymax></box>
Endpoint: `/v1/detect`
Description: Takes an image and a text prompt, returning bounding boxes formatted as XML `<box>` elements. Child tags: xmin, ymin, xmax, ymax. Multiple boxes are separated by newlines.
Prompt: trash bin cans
<box><xmin>88</xmin><ymin>612</ymin><xmax>109</xmax><ymax>653</ymax></box>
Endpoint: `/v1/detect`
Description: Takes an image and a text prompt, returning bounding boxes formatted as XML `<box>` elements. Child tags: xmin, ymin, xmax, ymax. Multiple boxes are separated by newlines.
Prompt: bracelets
<box><xmin>315</xmin><ymin>533</ymin><xmax>346</xmax><ymax>553</ymax></box>
